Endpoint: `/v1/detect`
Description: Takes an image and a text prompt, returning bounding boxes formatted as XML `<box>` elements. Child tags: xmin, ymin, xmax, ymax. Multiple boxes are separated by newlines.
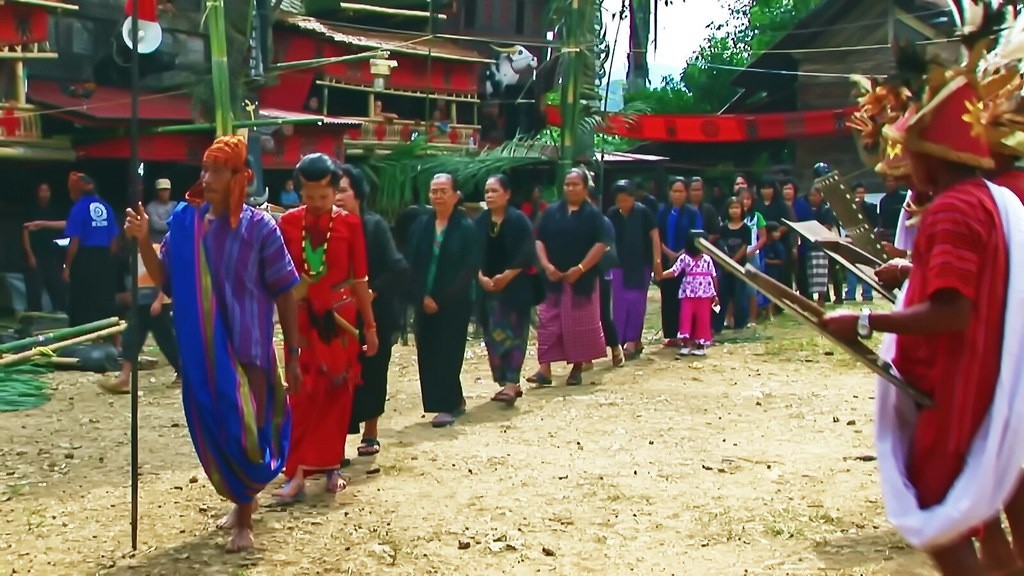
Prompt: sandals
<box><xmin>272</xmin><ymin>477</ymin><xmax>308</xmax><ymax>504</ymax></box>
<box><xmin>326</xmin><ymin>470</ymin><xmax>347</xmax><ymax>493</ymax></box>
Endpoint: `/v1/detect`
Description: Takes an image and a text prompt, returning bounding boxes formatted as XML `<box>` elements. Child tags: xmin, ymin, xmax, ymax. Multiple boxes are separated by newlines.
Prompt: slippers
<box><xmin>357</xmin><ymin>438</ymin><xmax>381</xmax><ymax>456</ymax></box>
<box><xmin>566</xmin><ymin>365</ymin><xmax>583</xmax><ymax>385</ymax></box>
<box><xmin>431</xmin><ymin>415</ymin><xmax>455</xmax><ymax>427</ymax></box>
<box><xmin>98</xmin><ymin>378</ymin><xmax>132</xmax><ymax>394</ymax></box>
<box><xmin>166</xmin><ymin>379</ymin><xmax>182</xmax><ymax>389</ymax></box>
<box><xmin>491</xmin><ymin>386</ymin><xmax>522</xmax><ymax>401</ymax></box>
<box><xmin>525</xmin><ymin>371</ymin><xmax>552</xmax><ymax>385</ymax></box>
<box><xmin>492</xmin><ymin>389</ymin><xmax>521</xmax><ymax>404</ymax></box>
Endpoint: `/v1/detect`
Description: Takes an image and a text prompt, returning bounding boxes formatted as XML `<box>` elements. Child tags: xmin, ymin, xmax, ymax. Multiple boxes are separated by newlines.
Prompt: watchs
<box><xmin>289</xmin><ymin>347</ymin><xmax>301</xmax><ymax>356</ymax></box>
<box><xmin>856</xmin><ymin>306</ymin><xmax>873</xmax><ymax>341</ymax></box>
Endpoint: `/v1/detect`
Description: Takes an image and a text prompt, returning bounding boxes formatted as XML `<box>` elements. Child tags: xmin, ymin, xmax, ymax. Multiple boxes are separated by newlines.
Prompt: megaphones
<box><xmin>122</xmin><ymin>16</ymin><xmax>163</xmax><ymax>54</ymax></box>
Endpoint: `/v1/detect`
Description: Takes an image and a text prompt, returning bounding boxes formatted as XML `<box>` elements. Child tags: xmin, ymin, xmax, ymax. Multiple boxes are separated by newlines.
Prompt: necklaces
<box><xmin>303</xmin><ymin>202</ymin><xmax>337</xmax><ymax>276</ymax></box>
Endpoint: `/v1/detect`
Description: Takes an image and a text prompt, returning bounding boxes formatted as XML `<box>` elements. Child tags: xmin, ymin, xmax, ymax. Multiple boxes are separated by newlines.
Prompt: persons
<box><xmin>581</xmin><ymin>61</ymin><xmax>1024</xmax><ymax>373</ymax></box>
<box><xmin>472</xmin><ymin>176</ymin><xmax>538</xmax><ymax>404</ymax></box>
<box><xmin>323</xmin><ymin>164</ymin><xmax>440</xmax><ymax>468</ymax></box>
<box><xmin>21</xmin><ymin>77</ymin><xmax>504</xmax><ymax>394</ymax></box>
<box><xmin>525</xmin><ymin>166</ymin><xmax>609</xmax><ymax>386</ymax></box>
<box><xmin>124</xmin><ymin>136</ymin><xmax>301</xmax><ymax>554</ymax></box>
<box><xmin>401</xmin><ymin>170</ymin><xmax>482</xmax><ymax>427</ymax></box>
<box><xmin>272</xmin><ymin>152</ymin><xmax>380</xmax><ymax>498</ymax></box>
<box><xmin>816</xmin><ymin>73</ymin><xmax>1024</xmax><ymax>576</ymax></box>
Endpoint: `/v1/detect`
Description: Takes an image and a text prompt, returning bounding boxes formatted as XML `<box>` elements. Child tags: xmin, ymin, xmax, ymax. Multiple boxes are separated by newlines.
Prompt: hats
<box><xmin>155</xmin><ymin>178</ymin><xmax>172</xmax><ymax>191</ymax></box>
<box><xmin>846</xmin><ymin>0</ymin><xmax>1023</xmax><ymax>176</ymax></box>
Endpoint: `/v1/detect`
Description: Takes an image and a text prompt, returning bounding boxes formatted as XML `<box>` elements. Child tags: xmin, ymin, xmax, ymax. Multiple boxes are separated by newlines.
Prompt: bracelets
<box><xmin>897</xmin><ymin>263</ymin><xmax>904</xmax><ymax>283</ymax></box>
<box><xmin>578</xmin><ymin>263</ymin><xmax>586</xmax><ymax>275</ymax></box>
<box><xmin>363</xmin><ymin>322</ymin><xmax>377</xmax><ymax>333</ymax></box>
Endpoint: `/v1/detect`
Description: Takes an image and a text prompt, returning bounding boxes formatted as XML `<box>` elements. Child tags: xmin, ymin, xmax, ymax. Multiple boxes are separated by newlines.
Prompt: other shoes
<box><xmin>608</xmin><ymin>287</ymin><xmax>875</xmax><ymax>366</ymax></box>
